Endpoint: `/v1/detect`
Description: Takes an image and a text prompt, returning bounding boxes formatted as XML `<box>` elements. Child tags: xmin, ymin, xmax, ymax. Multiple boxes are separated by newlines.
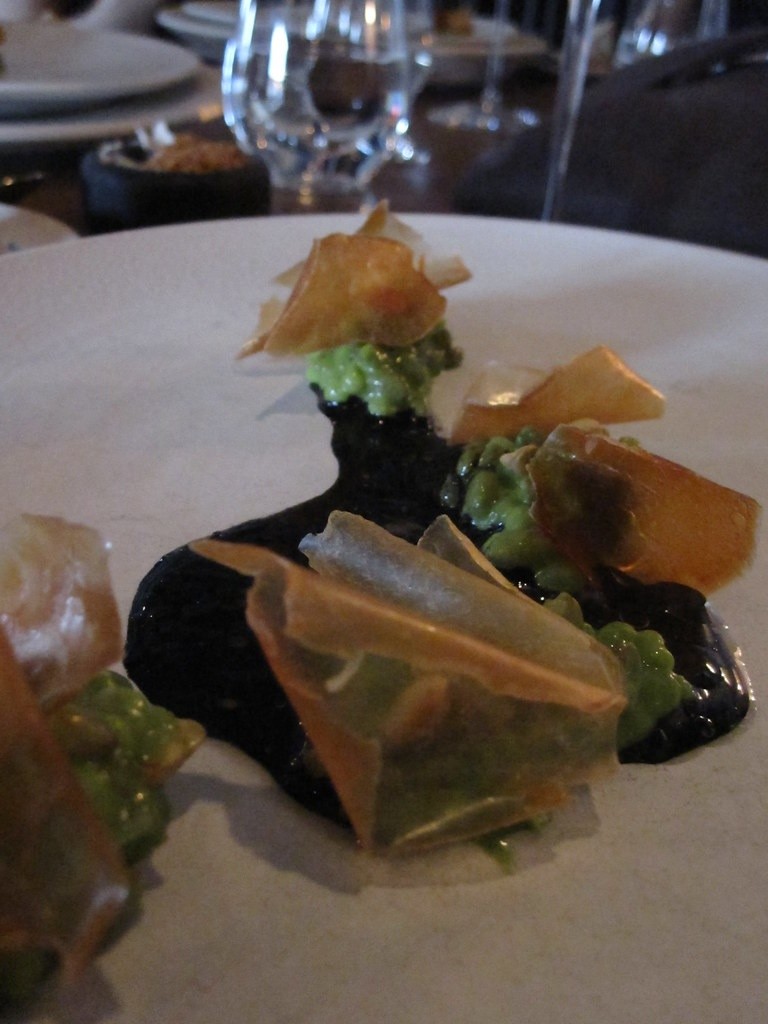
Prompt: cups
<box><xmin>217</xmin><ymin>0</ymin><xmax>434</xmax><ymax>219</ymax></box>
<box><xmin>518</xmin><ymin>0</ymin><xmax>753</xmax><ymax>244</ymax></box>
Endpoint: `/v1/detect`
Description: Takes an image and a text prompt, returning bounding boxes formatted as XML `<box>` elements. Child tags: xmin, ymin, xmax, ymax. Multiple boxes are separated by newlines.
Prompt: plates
<box><xmin>158</xmin><ymin>0</ymin><xmax>551</xmax><ymax>89</ymax></box>
<box><xmin>0</xmin><ymin>19</ymin><xmax>201</xmax><ymax>117</ymax></box>
<box><xmin>0</xmin><ymin>62</ymin><xmax>228</xmax><ymax>146</ymax></box>
<box><xmin>0</xmin><ymin>212</ymin><xmax>768</xmax><ymax>1024</ymax></box>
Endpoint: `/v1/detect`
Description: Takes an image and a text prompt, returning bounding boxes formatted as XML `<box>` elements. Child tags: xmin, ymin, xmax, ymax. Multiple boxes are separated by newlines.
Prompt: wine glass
<box><xmin>426</xmin><ymin>1</ymin><xmax>542</xmax><ymax>133</ymax></box>
